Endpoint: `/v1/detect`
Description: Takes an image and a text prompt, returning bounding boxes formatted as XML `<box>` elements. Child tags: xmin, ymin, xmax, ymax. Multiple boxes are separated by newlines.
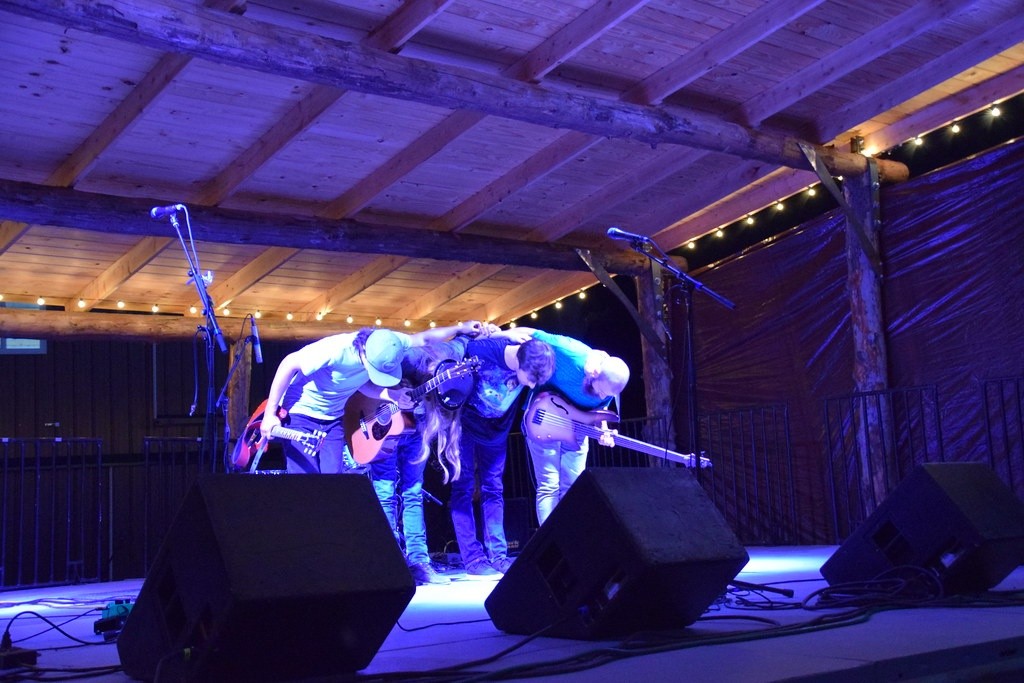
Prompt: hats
<box><xmin>366</xmin><ymin>330</ymin><xmax>404</xmax><ymax>387</ymax></box>
<box><xmin>433</xmin><ymin>359</ymin><xmax>474</xmax><ymax>412</ymax></box>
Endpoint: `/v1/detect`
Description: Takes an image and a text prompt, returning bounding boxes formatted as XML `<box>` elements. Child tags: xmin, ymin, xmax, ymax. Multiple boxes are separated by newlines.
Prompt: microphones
<box><xmin>251</xmin><ymin>316</ymin><xmax>263</xmax><ymax>363</ymax></box>
<box><xmin>607</xmin><ymin>228</ymin><xmax>650</xmax><ymax>243</ymax></box>
<box><xmin>151</xmin><ymin>204</ymin><xmax>183</xmax><ymax>220</ymax></box>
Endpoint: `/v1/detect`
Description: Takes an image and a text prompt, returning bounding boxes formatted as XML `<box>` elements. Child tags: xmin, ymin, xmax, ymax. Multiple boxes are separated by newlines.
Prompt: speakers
<box><xmin>484</xmin><ymin>466</ymin><xmax>750</xmax><ymax>641</ymax></box>
<box><xmin>116</xmin><ymin>472</ymin><xmax>417</xmax><ymax>683</ymax></box>
<box><xmin>820</xmin><ymin>462</ymin><xmax>1024</xmax><ymax>608</ymax></box>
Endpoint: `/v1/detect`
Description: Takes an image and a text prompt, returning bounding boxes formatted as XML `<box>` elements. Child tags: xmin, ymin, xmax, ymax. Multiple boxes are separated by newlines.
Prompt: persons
<box><xmin>259</xmin><ymin>321</ymin><xmax>629</xmax><ymax>585</ymax></box>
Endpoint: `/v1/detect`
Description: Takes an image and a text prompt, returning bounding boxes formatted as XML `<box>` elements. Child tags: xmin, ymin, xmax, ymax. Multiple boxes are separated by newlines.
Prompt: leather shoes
<box><xmin>409</xmin><ymin>562</ymin><xmax>451</xmax><ymax>586</ymax></box>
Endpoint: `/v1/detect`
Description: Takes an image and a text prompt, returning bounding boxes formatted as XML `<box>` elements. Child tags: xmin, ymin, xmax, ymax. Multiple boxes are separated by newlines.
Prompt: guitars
<box><xmin>231</xmin><ymin>396</ymin><xmax>328</xmax><ymax>470</ymax></box>
<box><xmin>525</xmin><ymin>390</ymin><xmax>713</xmax><ymax>470</ymax></box>
<box><xmin>343</xmin><ymin>354</ymin><xmax>481</xmax><ymax>465</ymax></box>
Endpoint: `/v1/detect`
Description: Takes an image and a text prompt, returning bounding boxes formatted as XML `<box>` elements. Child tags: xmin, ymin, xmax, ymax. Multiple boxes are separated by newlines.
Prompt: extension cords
<box><xmin>0</xmin><ymin>644</ymin><xmax>37</xmax><ymax>669</ymax></box>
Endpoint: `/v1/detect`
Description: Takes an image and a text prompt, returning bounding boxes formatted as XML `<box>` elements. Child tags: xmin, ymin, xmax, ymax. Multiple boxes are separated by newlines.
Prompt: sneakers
<box><xmin>491</xmin><ymin>557</ymin><xmax>513</xmax><ymax>575</ymax></box>
<box><xmin>466</xmin><ymin>559</ymin><xmax>504</xmax><ymax>581</ymax></box>
